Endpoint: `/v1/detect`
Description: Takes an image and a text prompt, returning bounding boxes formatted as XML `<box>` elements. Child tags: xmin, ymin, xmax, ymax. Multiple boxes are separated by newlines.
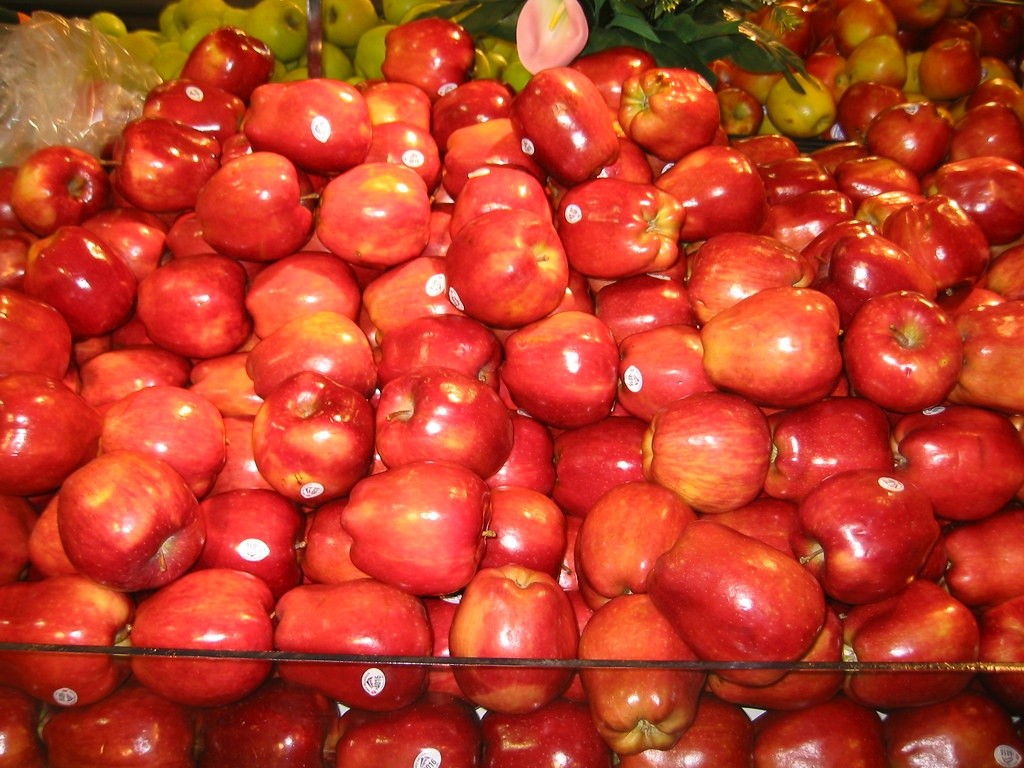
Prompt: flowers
<box><xmin>414</xmin><ymin>0</ymin><xmax>809</xmax><ymax>94</ymax></box>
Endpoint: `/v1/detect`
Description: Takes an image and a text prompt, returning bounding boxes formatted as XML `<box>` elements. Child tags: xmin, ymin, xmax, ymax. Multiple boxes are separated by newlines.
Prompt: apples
<box><xmin>0</xmin><ymin>0</ymin><xmax>1024</xmax><ymax>768</ymax></box>
